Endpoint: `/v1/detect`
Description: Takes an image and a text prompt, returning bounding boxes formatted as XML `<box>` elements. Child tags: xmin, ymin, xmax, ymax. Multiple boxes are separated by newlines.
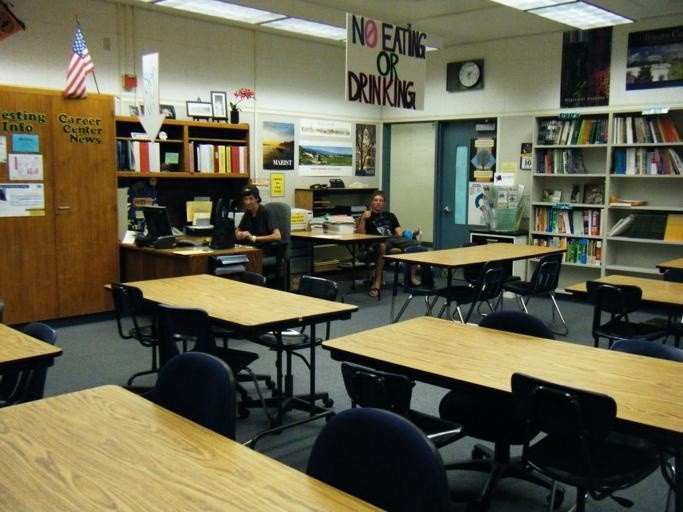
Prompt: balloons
<box><xmin>456</xmin><ymin>60</ymin><xmax>483</xmax><ymax>90</ymax></box>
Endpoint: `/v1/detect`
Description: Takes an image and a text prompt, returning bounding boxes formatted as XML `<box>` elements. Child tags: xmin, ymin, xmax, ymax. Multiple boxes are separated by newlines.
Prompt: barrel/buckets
<box><xmin>369</xmin><ymin>284</ymin><xmax>379</xmax><ymax>297</ymax></box>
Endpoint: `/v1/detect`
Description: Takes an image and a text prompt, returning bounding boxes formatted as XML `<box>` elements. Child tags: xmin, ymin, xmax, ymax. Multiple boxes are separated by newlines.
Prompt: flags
<box><xmin>230</xmin><ymin>108</ymin><xmax>239</xmax><ymax>124</ymax></box>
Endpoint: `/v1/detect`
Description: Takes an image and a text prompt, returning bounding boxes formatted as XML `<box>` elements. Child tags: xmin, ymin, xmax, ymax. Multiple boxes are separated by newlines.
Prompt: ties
<box><xmin>142</xmin><ymin>206</ymin><xmax>172</xmax><ymax>239</ymax></box>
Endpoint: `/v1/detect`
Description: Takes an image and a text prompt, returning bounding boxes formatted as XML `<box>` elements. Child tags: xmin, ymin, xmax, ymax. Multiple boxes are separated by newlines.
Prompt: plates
<box><xmin>524</xmin><ymin>105</ymin><xmax>683</xmax><ymax>299</ymax></box>
<box><xmin>288</xmin><ymin>185</ymin><xmax>383</xmax><ymax>274</ymax></box>
<box><xmin>0</xmin><ymin>83</ymin><xmax>126</xmax><ymax>328</ymax></box>
<box><xmin>113</xmin><ymin>115</ymin><xmax>251</xmax><ymax>180</ymax></box>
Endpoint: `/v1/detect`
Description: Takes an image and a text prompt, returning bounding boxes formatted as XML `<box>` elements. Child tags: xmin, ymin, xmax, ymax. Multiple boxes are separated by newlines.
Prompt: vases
<box><xmin>228</xmin><ymin>88</ymin><xmax>257</xmax><ymax>111</ymax></box>
<box><xmin>230</xmin><ymin>108</ymin><xmax>239</xmax><ymax>124</ymax></box>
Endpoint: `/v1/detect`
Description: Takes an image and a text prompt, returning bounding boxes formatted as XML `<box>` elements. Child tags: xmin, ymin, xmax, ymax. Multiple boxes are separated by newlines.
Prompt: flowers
<box><xmin>228</xmin><ymin>88</ymin><xmax>257</xmax><ymax>111</ymax></box>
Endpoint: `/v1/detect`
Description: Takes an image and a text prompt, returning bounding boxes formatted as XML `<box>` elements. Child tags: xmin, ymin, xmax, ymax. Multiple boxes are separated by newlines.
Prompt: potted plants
<box><xmin>139</xmin><ymin>104</ymin><xmax>176</xmax><ymax>120</ymax></box>
<box><xmin>184</xmin><ymin>99</ymin><xmax>212</xmax><ymax>123</ymax></box>
<box><xmin>208</xmin><ymin>90</ymin><xmax>228</xmax><ymax>124</ymax></box>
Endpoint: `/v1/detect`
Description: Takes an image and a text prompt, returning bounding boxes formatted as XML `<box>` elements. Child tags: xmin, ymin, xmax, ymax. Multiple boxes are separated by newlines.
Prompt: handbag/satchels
<box><xmin>249</xmin><ymin>235</ymin><xmax>257</xmax><ymax>243</ymax></box>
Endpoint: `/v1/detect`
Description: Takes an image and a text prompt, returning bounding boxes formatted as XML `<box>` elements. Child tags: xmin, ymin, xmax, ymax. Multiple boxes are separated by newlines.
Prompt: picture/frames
<box><xmin>0</xmin><ymin>382</ymin><xmax>384</xmax><ymax>511</ymax></box>
<box><xmin>208</xmin><ymin>90</ymin><xmax>228</xmax><ymax>124</ymax></box>
<box><xmin>139</xmin><ymin>104</ymin><xmax>176</xmax><ymax>120</ymax></box>
<box><xmin>0</xmin><ymin>320</ymin><xmax>62</xmax><ymax>406</ymax></box>
<box><xmin>184</xmin><ymin>99</ymin><xmax>212</xmax><ymax>123</ymax></box>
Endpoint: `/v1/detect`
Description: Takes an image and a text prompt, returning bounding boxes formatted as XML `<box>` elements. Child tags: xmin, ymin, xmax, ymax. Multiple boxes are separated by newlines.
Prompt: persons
<box><xmin>356</xmin><ymin>191</ymin><xmax>422</xmax><ymax>297</ymax></box>
<box><xmin>233</xmin><ymin>185</ymin><xmax>282</xmax><ymax>275</ymax></box>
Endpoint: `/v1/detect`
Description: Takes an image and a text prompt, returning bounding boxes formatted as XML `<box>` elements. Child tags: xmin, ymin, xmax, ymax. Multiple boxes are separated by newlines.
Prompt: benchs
<box><xmin>63</xmin><ymin>23</ymin><xmax>96</xmax><ymax>100</ymax></box>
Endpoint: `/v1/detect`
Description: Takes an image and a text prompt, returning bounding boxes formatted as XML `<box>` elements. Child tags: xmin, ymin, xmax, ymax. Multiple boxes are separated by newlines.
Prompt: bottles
<box><xmin>240</xmin><ymin>185</ymin><xmax>260</xmax><ymax>203</ymax></box>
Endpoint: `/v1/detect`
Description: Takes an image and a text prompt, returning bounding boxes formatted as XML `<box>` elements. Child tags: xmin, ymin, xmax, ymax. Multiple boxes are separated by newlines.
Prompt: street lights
<box><xmin>210</xmin><ymin>198</ymin><xmax>235</xmax><ymax>250</ymax></box>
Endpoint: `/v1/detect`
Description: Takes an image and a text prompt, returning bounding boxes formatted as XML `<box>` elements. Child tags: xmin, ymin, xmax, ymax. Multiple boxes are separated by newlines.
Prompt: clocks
<box><xmin>456</xmin><ymin>60</ymin><xmax>483</xmax><ymax>90</ymax></box>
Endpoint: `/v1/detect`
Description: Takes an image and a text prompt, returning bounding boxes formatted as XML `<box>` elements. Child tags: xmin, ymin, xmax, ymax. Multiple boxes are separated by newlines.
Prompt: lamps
<box><xmin>0</xmin><ymin>321</ymin><xmax>59</xmax><ymax>407</ymax></box>
<box><xmin>490</xmin><ymin>0</ymin><xmax>644</xmax><ymax>34</ymax></box>
<box><xmin>145</xmin><ymin>0</ymin><xmax>444</xmax><ymax>57</ymax></box>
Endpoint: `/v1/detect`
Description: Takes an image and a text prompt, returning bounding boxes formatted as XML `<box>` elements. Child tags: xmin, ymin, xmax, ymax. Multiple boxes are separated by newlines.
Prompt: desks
<box><xmin>322</xmin><ymin>317</ymin><xmax>682</xmax><ymax>510</ymax></box>
<box><xmin>565</xmin><ymin>258</ymin><xmax>682</xmax><ymax>350</ymax></box>
<box><xmin>0</xmin><ymin>320</ymin><xmax>62</xmax><ymax>406</ymax></box>
<box><xmin>0</xmin><ymin>382</ymin><xmax>384</xmax><ymax>511</ymax></box>
<box><xmin>120</xmin><ymin>232</ymin><xmax>393</xmax><ymax>296</ymax></box>
<box><xmin>103</xmin><ymin>273</ymin><xmax>359</xmax><ymax>450</ymax></box>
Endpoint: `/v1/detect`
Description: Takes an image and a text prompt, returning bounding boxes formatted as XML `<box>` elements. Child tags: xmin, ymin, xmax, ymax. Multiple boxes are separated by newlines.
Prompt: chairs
<box><xmin>0</xmin><ymin>321</ymin><xmax>59</xmax><ymax>407</ymax></box>
<box><xmin>106</xmin><ymin>271</ymin><xmax>340</xmax><ymax>418</ymax></box>
<box><xmin>307</xmin><ymin>408</ymin><xmax>451</xmax><ymax>512</ymax></box>
<box><xmin>342</xmin><ymin>311</ymin><xmax>682</xmax><ymax>510</ymax></box>
<box><xmin>381</xmin><ymin>232</ymin><xmax>421</xmax><ymax>289</ymax></box>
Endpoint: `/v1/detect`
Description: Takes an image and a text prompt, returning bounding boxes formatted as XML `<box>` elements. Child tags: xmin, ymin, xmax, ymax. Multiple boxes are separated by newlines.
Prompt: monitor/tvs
<box><xmin>532</xmin><ymin>110</ymin><xmax>682</xmax><ymax>268</ymax></box>
<box><xmin>309</xmin><ymin>213</ymin><xmax>355</xmax><ymax>234</ymax></box>
<box><xmin>115</xmin><ymin>140</ymin><xmax>249</xmax><ymax>174</ymax></box>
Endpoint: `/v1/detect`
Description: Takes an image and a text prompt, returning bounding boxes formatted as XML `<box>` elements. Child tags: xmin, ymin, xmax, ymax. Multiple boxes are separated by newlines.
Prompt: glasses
<box><xmin>145</xmin><ymin>0</ymin><xmax>444</xmax><ymax>57</ymax></box>
<box><xmin>490</xmin><ymin>0</ymin><xmax>644</xmax><ymax>34</ymax></box>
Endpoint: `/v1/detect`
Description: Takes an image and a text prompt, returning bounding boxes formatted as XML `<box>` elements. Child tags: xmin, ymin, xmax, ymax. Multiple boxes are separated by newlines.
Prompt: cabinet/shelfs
<box><xmin>288</xmin><ymin>185</ymin><xmax>383</xmax><ymax>274</ymax></box>
<box><xmin>113</xmin><ymin>115</ymin><xmax>251</xmax><ymax>180</ymax></box>
<box><xmin>524</xmin><ymin>105</ymin><xmax>683</xmax><ymax>299</ymax></box>
<box><xmin>0</xmin><ymin>83</ymin><xmax>126</xmax><ymax>328</ymax></box>
